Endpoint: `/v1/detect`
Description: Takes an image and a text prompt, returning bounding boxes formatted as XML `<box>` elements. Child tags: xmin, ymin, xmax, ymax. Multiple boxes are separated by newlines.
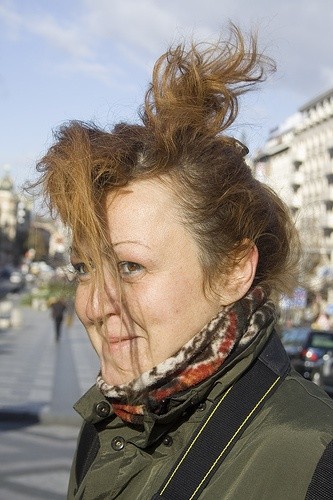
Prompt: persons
<box><xmin>22</xmin><ymin>20</ymin><xmax>332</xmax><ymax>499</ymax></box>
<box><xmin>49</xmin><ymin>297</ymin><xmax>66</xmax><ymax>345</ymax></box>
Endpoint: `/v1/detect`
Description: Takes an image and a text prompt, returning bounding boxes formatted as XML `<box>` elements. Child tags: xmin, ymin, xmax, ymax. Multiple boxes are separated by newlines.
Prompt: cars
<box><xmin>0</xmin><ymin>259</ymin><xmax>74</xmax><ymax>299</ymax></box>
<box><xmin>275</xmin><ymin>324</ymin><xmax>333</xmax><ymax>398</ymax></box>
